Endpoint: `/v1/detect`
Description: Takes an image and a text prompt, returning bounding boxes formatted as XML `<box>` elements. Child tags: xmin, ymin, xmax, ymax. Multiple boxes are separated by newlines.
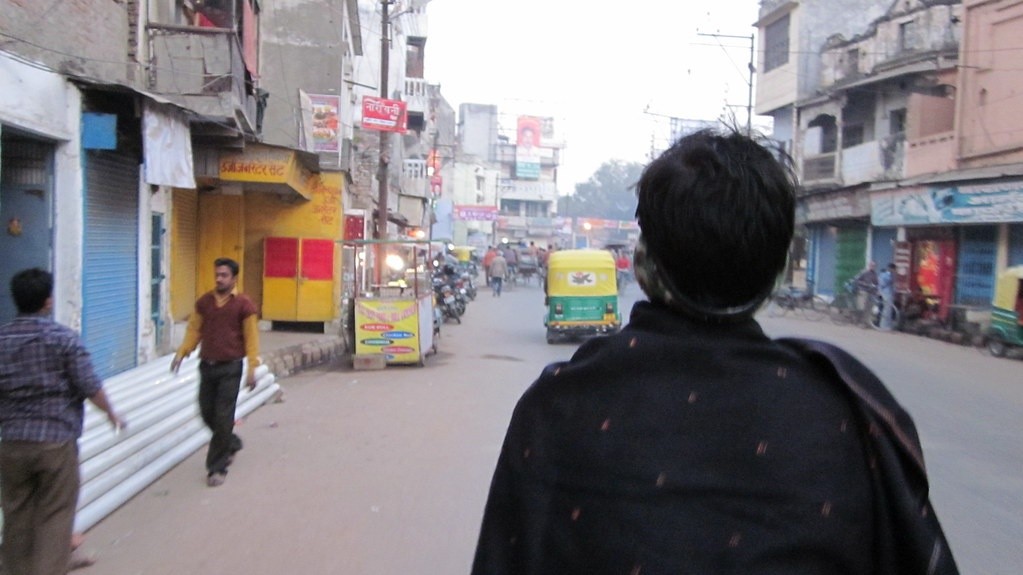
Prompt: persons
<box><xmin>855</xmin><ymin>262</ymin><xmax>925</xmax><ymax>331</ymax></box>
<box><xmin>170</xmin><ymin>257</ymin><xmax>261</xmax><ymax>485</ymax></box>
<box><xmin>0</xmin><ymin>268</ymin><xmax>127</xmax><ymax>575</ymax></box>
<box><xmin>471</xmin><ymin>122</ymin><xmax>961</xmax><ymax>575</ymax></box>
<box><xmin>418</xmin><ymin>240</ymin><xmax>563</xmax><ymax>297</ymax></box>
<box><xmin>614</xmin><ymin>248</ymin><xmax>633</xmax><ymax>290</ymax></box>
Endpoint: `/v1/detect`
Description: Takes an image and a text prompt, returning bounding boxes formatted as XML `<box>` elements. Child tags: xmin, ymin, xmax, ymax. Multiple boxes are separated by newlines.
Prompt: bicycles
<box><xmin>770</xmin><ymin>278</ymin><xmax>827</xmax><ymax>322</ymax></box>
<box><xmin>828</xmin><ymin>283</ymin><xmax>901</xmax><ymax>333</ymax></box>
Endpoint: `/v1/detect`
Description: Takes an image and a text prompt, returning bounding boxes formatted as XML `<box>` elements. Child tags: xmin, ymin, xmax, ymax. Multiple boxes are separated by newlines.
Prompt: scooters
<box><xmin>428</xmin><ymin>243</ymin><xmax>478</xmax><ymax>325</ymax></box>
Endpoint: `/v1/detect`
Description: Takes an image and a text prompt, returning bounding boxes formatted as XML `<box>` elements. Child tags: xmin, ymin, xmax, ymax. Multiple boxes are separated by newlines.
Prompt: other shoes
<box><xmin>207</xmin><ymin>465</ymin><xmax>228</xmax><ymax>486</ymax></box>
<box><xmin>226</xmin><ymin>439</ymin><xmax>243</xmax><ymax>466</ymax></box>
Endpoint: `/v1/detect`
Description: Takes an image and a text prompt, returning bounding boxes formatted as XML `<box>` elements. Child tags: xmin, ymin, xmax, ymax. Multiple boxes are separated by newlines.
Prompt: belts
<box><xmin>202</xmin><ymin>358</ymin><xmax>233</xmax><ymax>367</ymax></box>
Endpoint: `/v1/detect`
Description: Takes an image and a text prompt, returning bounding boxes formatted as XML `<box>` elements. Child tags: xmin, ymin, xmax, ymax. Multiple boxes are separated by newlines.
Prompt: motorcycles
<box><xmin>449</xmin><ymin>244</ymin><xmax>482</xmax><ymax>265</ymax></box>
<box><xmin>542</xmin><ymin>249</ymin><xmax>622</xmax><ymax>345</ymax></box>
<box><xmin>986</xmin><ymin>265</ymin><xmax>1023</xmax><ymax>358</ymax></box>
